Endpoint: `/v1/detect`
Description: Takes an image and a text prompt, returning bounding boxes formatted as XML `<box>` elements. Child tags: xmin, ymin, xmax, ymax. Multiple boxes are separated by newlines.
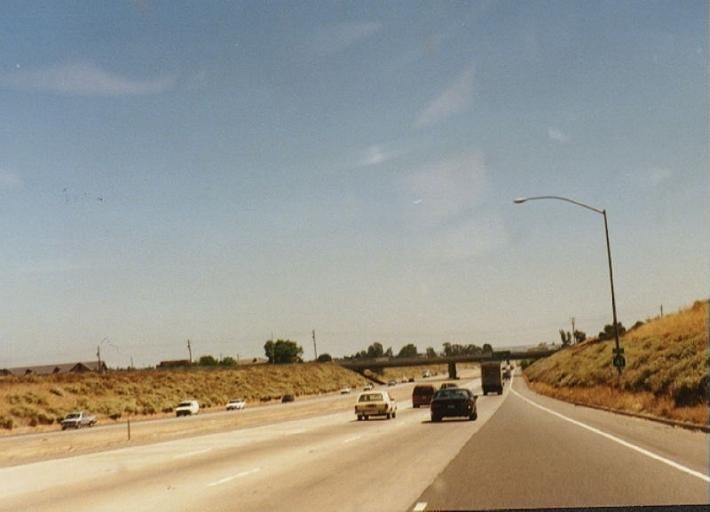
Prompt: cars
<box><xmin>225</xmin><ymin>399</ymin><xmax>247</xmax><ymax>410</ymax></box>
<box><xmin>61</xmin><ymin>411</ymin><xmax>98</xmax><ymax>429</ymax></box>
<box><xmin>440</xmin><ymin>383</ymin><xmax>457</xmax><ymax>389</ymax></box>
<box><xmin>355</xmin><ymin>391</ymin><xmax>397</xmax><ymax>419</ymax></box>
<box><xmin>339</xmin><ymin>385</ymin><xmax>352</xmax><ymax>394</ymax></box>
<box><xmin>280</xmin><ymin>394</ymin><xmax>296</xmax><ymax>403</ymax></box>
<box><xmin>362</xmin><ymin>369</ymin><xmax>440</xmax><ymax>390</ymax></box>
<box><xmin>430</xmin><ymin>387</ymin><xmax>479</xmax><ymax>421</ymax></box>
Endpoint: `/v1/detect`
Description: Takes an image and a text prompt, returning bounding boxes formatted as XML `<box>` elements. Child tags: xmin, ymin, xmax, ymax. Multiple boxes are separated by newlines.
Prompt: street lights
<box><xmin>513</xmin><ymin>195</ymin><xmax>622</xmax><ymax>375</ymax></box>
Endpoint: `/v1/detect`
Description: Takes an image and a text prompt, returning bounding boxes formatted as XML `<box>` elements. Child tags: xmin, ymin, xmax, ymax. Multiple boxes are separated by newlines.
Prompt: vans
<box><xmin>175</xmin><ymin>399</ymin><xmax>200</xmax><ymax>416</ymax></box>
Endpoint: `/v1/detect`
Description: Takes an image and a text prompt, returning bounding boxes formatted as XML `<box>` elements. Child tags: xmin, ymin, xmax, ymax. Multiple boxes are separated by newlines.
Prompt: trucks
<box><xmin>482</xmin><ymin>362</ymin><xmax>503</xmax><ymax>395</ymax></box>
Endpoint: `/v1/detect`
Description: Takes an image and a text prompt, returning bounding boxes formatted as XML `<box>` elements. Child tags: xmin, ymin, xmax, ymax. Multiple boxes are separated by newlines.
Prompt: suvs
<box><xmin>413</xmin><ymin>384</ymin><xmax>437</xmax><ymax>408</ymax></box>
